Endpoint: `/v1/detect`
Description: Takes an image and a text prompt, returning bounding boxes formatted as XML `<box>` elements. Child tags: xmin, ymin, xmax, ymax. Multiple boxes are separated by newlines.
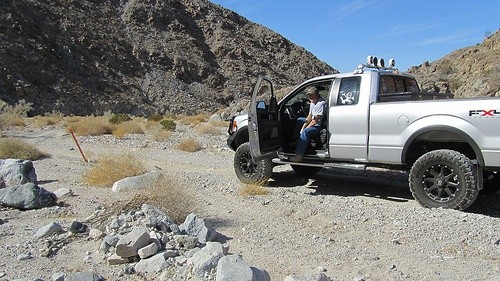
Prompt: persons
<box><xmin>287</xmin><ymin>87</ymin><xmax>328</xmax><ymax>163</ymax></box>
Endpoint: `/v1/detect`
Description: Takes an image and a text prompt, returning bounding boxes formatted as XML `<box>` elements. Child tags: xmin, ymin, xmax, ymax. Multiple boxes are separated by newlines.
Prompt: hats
<box><xmin>305</xmin><ymin>87</ymin><xmax>319</xmax><ymax>95</ymax></box>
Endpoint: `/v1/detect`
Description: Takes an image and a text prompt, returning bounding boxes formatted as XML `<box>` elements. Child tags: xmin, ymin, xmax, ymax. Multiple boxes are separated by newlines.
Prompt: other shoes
<box><xmin>289</xmin><ymin>155</ymin><xmax>303</xmax><ymax>162</ymax></box>
<box><xmin>289</xmin><ymin>140</ymin><xmax>298</xmax><ymax>145</ymax></box>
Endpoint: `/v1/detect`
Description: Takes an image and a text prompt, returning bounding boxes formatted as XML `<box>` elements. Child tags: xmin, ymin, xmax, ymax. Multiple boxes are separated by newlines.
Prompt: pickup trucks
<box><xmin>227</xmin><ymin>55</ymin><xmax>499</xmax><ymax>210</ymax></box>
